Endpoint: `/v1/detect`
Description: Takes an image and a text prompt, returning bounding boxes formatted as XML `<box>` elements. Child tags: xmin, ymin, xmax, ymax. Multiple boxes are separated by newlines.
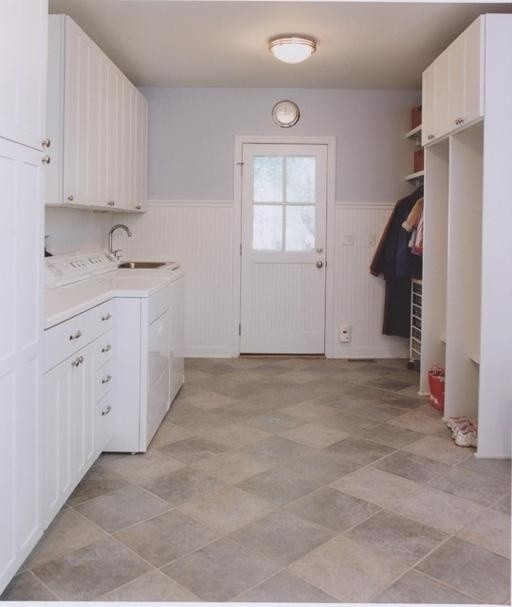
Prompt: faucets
<box><xmin>106</xmin><ymin>223</ymin><xmax>133</xmax><ymax>259</ymax></box>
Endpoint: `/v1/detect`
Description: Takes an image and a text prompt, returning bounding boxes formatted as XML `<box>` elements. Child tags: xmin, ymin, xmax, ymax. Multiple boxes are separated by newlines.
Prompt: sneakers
<box><xmin>441</xmin><ymin>414</ymin><xmax>477</xmax><ymax>446</ymax></box>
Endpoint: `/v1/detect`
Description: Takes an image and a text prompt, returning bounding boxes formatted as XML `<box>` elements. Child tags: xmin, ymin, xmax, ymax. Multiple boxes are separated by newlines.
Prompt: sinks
<box><xmin>118</xmin><ymin>259</ymin><xmax>165</xmax><ymax>271</ymax></box>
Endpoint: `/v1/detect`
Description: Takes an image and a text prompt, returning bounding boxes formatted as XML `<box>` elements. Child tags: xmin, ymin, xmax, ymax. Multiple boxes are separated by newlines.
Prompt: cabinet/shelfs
<box><xmin>104</xmin><ymin>274</ymin><xmax>185</xmax><ymax>455</ymax></box>
<box><xmin>42</xmin><ymin>308</ymin><xmax>93</xmax><ymax>532</ymax></box>
<box><xmin>403</xmin><ymin>10</ymin><xmax>512</xmax><ymax>461</ymax></box>
<box><xmin>0</xmin><ymin>0</ymin><xmax>50</xmax><ymax>596</ymax></box>
<box><xmin>51</xmin><ymin>13</ymin><xmax>148</xmax><ymax>213</ymax></box>
<box><xmin>93</xmin><ymin>299</ymin><xmax>114</xmax><ymax>462</ymax></box>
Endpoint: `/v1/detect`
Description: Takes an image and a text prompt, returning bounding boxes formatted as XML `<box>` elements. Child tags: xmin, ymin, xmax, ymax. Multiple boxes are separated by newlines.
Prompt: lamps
<box><xmin>268</xmin><ymin>35</ymin><xmax>316</xmax><ymax>64</ymax></box>
